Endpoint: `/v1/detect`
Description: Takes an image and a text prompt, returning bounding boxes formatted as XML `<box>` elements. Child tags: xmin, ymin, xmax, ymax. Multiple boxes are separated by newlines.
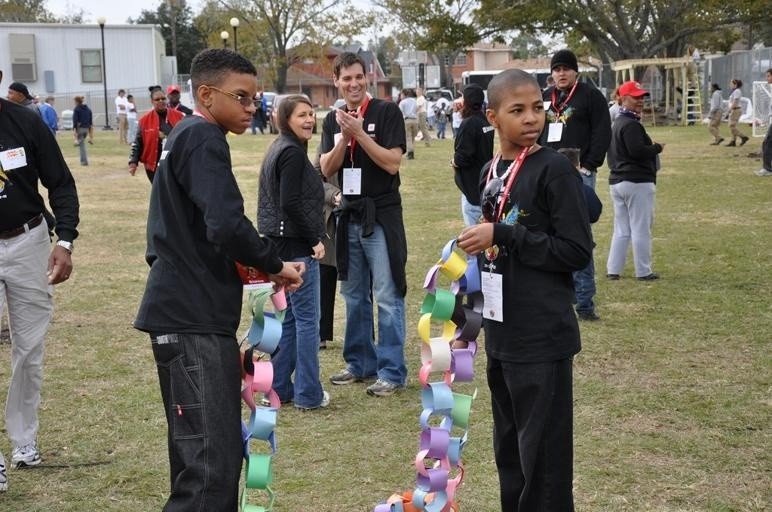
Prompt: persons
<box><xmin>256</xmin><ymin>95</ymin><xmax>332</xmax><ymax>412</ymax></box>
<box><xmin>72</xmin><ymin>95</ymin><xmax>93</xmax><ymax>165</ymax></box>
<box><xmin>449</xmin><ymin>66</ymin><xmax>596</xmax><ymax>512</ymax></box>
<box><xmin>115</xmin><ymin>85</ymin><xmax>192</xmax><ymax>185</ymax></box>
<box><xmin>450</xmin><ymin>87</ymin><xmax>496</xmax><ymax>292</ymax></box>
<box><xmin>1</xmin><ymin>70</ymin><xmax>81</xmax><ymax>497</ymax></box>
<box><xmin>675</xmin><ymin>79</ymin><xmax>749</xmax><ymax>147</ymax></box>
<box><xmin>607</xmin><ymin>80</ymin><xmax>666</xmax><ymax>280</ymax></box>
<box><xmin>130</xmin><ymin>48</ymin><xmax>308</xmax><ymax>512</ymax></box>
<box><xmin>535</xmin><ymin>49</ymin><xmax>613</xmax><ymax>320</ymax></box>
<box><xmin>317</xmin><ymin>51</ymin><xmax>409</xmax><ymax>398</ymax></box>
<box><xmin>754</xmin><ymin>69</ymin><xmax>772</xmax><ymax>178</ymax></box>
<box><xmin>312</xmin><ymin>140</ymin><xmax>342</xmax><ymax>350</ymax></box>
<box><xmin>4</xmin><ymin>82</ymin><xmax>59</xmax><ymax>135</ymax></box>
<box><xmin>608</xmin><ymin>88</ymin><xmax>626</xmax><ymax>127</ymax></box>
<box><xmin>398</xmin><ymin>87</ymin><xmax>467</xmax><ymax>160</ymax></box>
<box><xmin>250</xmin><ymin>93</ymin><xmax>269</xmax><ymax>136</ymax></box>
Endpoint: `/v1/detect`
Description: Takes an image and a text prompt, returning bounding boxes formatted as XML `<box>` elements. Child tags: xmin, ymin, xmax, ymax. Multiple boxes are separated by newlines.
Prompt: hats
<box><xmin>10</xmin><ymin>81</ymin><xmax>35</xmax><ymax>103</ymax></box>
<box><xmin>459</xmin><ymin>80</ymin><xmax>486</xmax><ymax>110</ymax></box>
<box><xmin>551</xmin><ymin>50</ymin><xmax>578</xmax><ymax>72</ymax></box>
<box><xmin>167</xmin><ymin>86</ymin><xmax>179</xmax><ymax>94</ymax></box>
<box><xmin>618</xmin><ymin>81</ymin><xmax>650</xmax><ymax>97</ymax></box>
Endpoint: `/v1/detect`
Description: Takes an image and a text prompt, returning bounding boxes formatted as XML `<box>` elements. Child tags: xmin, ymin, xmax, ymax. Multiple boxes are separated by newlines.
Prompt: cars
<box><xmin>255</xmin><ymin>90</ymin><xmax>279</xmax><ymax>121</ymax></box>
<box><xmin>268</xmin><ymin>94</ymin><xmax>318</xmax><ymax>135</ymax></box>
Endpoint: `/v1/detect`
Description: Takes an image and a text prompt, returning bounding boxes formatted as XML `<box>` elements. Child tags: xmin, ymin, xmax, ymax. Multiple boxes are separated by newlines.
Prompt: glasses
<box><xmin>207</xmin><ymin>85</ymin><xmax>263</xmax><ymax>109</ymax></box>
<box><xmin>481</xmin><ymin>178</ymin><xmax>503</xmax><ymax>222</ymax></box>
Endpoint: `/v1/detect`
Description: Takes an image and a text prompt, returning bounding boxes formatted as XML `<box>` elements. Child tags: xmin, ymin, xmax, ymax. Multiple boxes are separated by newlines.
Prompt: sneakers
<box><xmin>366</xmin><ymin>378</ymin><xmax>405</xmax><ymax>396</ymax></box>
<box><xmin>10</xmin><ymin>440</ymin><xmax>40</xmax><ymax>469</ymax></box>
<box><xmin>261</xmin><ymin>388</ymin><xmax>329</xmax><ymax>411</ymax></box>
<box><xmin>754</xmin><ymin>169</ymin><xmax>772</xmax><ymax>176</ymax></box>
<box><xmin>579</xmin><ymin>312</ymin><xmax>599</xmax><ymax>321</ymax></box>
<box><xmin>607</xmin><ymin>274</ymin><xmax>620</xmax><ymax>280</ymax></box>
<box><xmin>638</xmin><ymin>273</ymin><xmax>659</xmax><ymax>280</ymax></box>
<box><xmin>329</xmin><ymin>370</ymin><xmax>377</xmax><ymax>386</ymax></box>
<box><xmin>0</xmin><ymin>450</ymin><xmax>9</xmax><ymax>492</ymax></box>
<box><xmin>711</xmin><ymin>136</ymin><xmax>748</xmax><ymax>146</ymax></box>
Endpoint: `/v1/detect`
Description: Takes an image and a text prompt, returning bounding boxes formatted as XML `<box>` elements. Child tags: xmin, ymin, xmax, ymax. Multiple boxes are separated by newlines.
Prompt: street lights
<box><xmin>230</xmin><ymin>17</ymin><xmax>241</xmax><ymax>54</ymax></box>
<box><xmin>221</xmin><ymin>31</ymin><xmax>229</xmax><ymax>48</ymax></box>
<box><xmin>96</xmin><ymin>15</ymin><xmax>114</xmax><ymax>131</ymax></box>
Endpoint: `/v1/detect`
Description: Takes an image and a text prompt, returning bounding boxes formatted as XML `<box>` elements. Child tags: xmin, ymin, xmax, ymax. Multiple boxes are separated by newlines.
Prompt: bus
<box><xmin>458</xmin><ymin>67</ymin><xmax>608</xmax><ymax>108</ymax></box>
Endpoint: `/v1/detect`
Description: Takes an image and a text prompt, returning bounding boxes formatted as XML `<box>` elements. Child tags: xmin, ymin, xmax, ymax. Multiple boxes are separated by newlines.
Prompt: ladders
<box><xmin>681</xmin><ymin>64</ymin><xmax>702</xmax><ymax>127</ymax></box>
<box><xmin>639</xmin><ymin>83</ymin><xmax>656</xmax><ymax>127</ymax></box>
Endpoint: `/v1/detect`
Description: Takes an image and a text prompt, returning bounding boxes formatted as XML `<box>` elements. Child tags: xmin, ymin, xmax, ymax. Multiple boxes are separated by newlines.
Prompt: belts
<box><xmin>0</xmin><ymin>213</ymin><xmax>43</xmax><ymax>239</ymax></box>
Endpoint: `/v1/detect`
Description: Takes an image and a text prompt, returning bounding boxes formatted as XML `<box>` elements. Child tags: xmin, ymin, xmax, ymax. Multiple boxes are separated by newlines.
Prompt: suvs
<box><xmin>422</xmin><ymin>88</ymin><xmax>454</xmax><ymax>121</ymax></box>
